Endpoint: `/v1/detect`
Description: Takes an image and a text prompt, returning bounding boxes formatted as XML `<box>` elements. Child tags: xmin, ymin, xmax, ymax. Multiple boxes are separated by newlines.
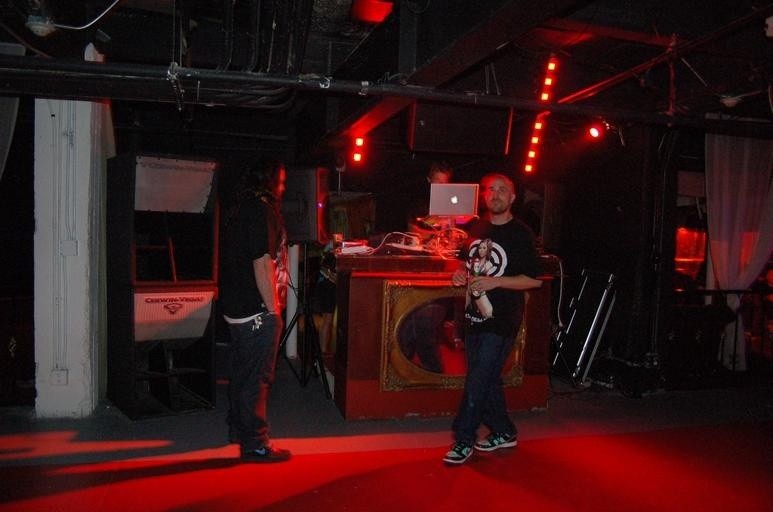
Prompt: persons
<box><xmin>217</xmin><ymin>162</ymin><xmax>290</xmax><ymax>463</ymax></box>
<box><xmin>442</xmin><ymin>174</ymin><xmax>543</xmax><ymax>463</ymax></box>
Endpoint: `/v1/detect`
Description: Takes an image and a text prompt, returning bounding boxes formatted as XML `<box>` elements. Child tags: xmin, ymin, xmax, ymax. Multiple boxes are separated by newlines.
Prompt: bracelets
<box><xmin>268</xmin><ymin>311</ymin><xmax>277</xmax><ymax>313</ymax></box>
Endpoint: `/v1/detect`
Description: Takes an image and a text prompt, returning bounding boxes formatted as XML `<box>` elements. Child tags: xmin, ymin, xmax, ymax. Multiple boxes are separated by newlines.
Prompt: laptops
<box><xmin>423</xmin><ymin>182</ymin><xmax>480</xmax><ymax>221</ymax></box>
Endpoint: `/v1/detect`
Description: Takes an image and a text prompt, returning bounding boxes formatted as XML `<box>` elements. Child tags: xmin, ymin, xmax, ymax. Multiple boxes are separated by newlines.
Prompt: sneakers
<box><xmin>228</xmin><ymin>427</ymin><xmax>291</xmax><ymax>464</ymax></box>
<box><xmin>442</xmin><ymin>443</ymin><xmax>475</xmax><ymax>466</ymax></box>
<box><xmin>473</xmin><ymin>431</ymin><xmax>518</xmax><ymax>452</ymax></box>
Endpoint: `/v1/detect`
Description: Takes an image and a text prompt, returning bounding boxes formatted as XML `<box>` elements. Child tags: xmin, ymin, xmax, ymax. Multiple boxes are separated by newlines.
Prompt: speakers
<box><xmin>411</xmin><ymin>99</ymin><xmax>515</xmax><ymax>156</ymax></box>
<box><xmin>283</xmin><ymin>168</ymin><xmax>333</xmax><ymax>244</ymax></box>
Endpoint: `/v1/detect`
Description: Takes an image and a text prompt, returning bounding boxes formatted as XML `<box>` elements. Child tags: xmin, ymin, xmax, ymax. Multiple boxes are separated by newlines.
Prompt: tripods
<box><xmin>280</xmin><ymin>243</ymin><xmax>337</xmax><ymax>401</ymax></box>
